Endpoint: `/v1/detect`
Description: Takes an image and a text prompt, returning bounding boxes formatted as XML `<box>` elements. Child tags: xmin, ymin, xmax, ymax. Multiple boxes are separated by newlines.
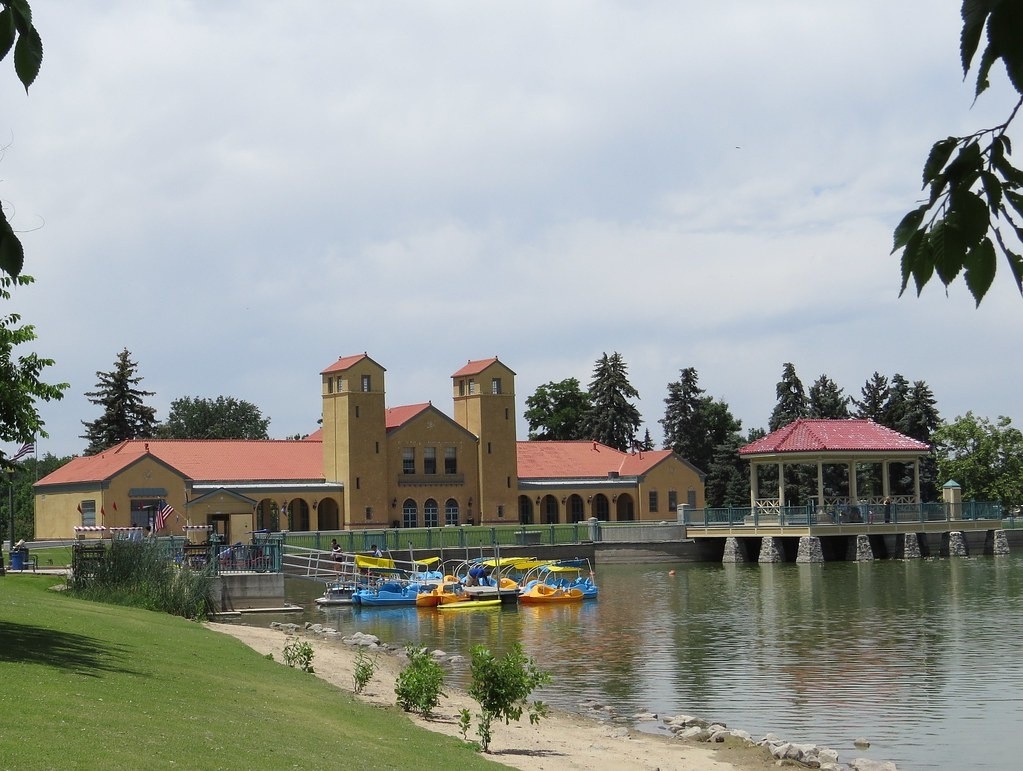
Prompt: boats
<box><xmin>313</xmin><ymin>553</ymin><xmax>599</xmax><ymax>610</ymax></box>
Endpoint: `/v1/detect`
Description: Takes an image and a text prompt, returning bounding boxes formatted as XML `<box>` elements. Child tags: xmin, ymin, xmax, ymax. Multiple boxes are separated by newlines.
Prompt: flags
<box><xmin>112</xmin><ymin>502</ymin><xmax>118</xmax><ymax>511</ymax></box>
<box><xmin>139</xmin><ymin>501</ymin><xmax>143</xmax><ymax>509</ymax></box>
<box><xmin>100</xmin><ymin>506</ymin><xmax>105</xmax><ymax>516</ymax></box>
<box><xmin>77</xmin><ymin>505</ymin><xmax>82</xmax><ymax>514</ymax></box>
<box><xmin>154</xmin><ymin>499</ymin><xmax>174</xmax><ymax>532</ymax></box>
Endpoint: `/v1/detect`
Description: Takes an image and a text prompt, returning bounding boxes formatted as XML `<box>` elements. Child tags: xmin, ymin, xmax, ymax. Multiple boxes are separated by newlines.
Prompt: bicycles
<box><xmin>221</xmin><ymin>547</ymin><xmax>268</xmax><ymax>574</ymax></box>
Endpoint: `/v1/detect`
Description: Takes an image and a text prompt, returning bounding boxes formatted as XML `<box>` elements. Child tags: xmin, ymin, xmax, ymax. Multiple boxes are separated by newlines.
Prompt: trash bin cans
<box><xmin>13</xmin><ymin>547</ymin><xmax>29</xmax><ymax>568</ymax></box>
<box><xmin>10</xmin><ymin>551</ymin><xmax>25</xmax><ymax>568</ymax></box>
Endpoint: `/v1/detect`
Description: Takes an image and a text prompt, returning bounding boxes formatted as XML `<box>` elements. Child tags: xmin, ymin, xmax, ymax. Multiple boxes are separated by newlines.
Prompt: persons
<box><xmin>668</xmin><ymin>569</ymin><xmax>675</xmax><ymax>576</ymax></box>
<box><xmin>143</xmin><ymin>526</ymin><xmax>154</xmax><ymax>539</ymax></box>
<box><xmin>371</xmin><ymin>544</ymin><xmax>383</xmax><ymax>558</ymax></box>
<box><xmin>826</xmin><ymin>500</ymin><xmax>837</xmax><ymax>523</ymax></box>
<box><xmin>463</xmin><ymin>568</ymin><xmax>490</xmax><ymax>587</ymax></box>
<box><xmin>883</xmin><ymin>497</ymin><xmax>891</xmax><ymax>523</ymax></box>
<box><xmin>328</xmin><ymin>538</ymin><xmax>345</xmax><ymax>581</ymax></box>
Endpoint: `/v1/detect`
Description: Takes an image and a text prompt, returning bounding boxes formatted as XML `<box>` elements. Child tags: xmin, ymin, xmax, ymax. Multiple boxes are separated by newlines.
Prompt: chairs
<box><xmin>19</xmin><ymin>549</ymin><xmax>39</xmax><ymax>574</ymax></box>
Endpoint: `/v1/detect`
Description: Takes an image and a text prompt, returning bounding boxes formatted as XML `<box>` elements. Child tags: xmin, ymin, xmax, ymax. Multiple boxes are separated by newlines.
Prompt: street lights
<box><xmin>6</xmin><ymin>456</ymin><xmax>17</xmax><ymax>553</ymax></box>
